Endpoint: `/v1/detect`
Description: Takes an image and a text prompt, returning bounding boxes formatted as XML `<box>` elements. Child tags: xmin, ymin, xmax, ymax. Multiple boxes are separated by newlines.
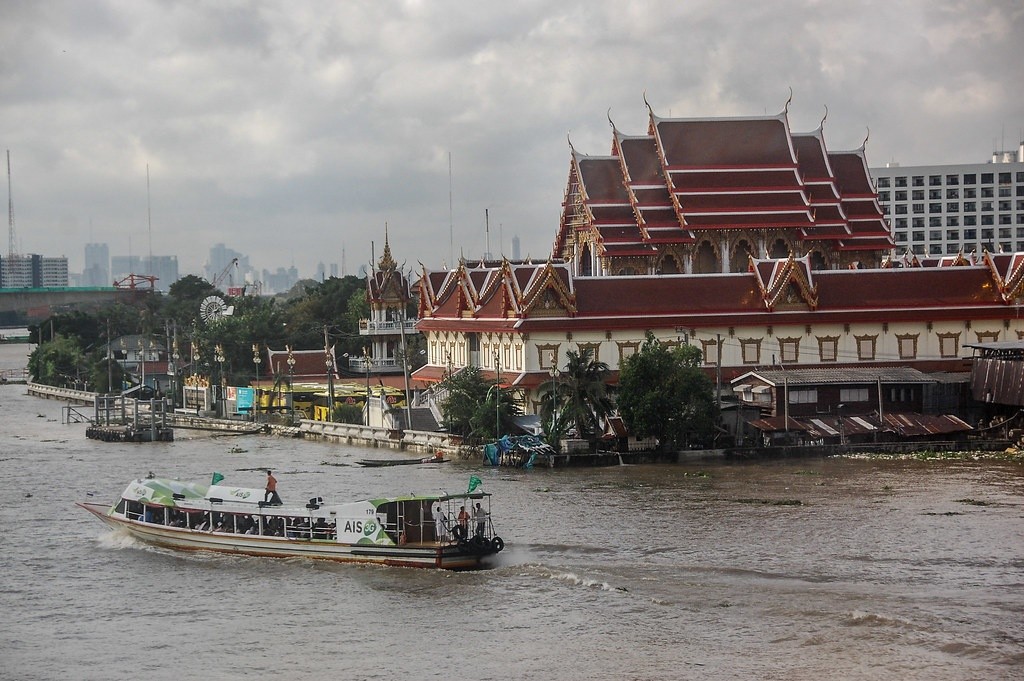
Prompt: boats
<box><xmin>118</xmin><ymin>337</ymin><xmax>425</xmax><ymax>430</ymax></box>
<box><xmin>357</xmin><ymin>453</ymin><xmax>451</xmax><ymax>467</ymax></box>
<box><xmin>76</xmin><ymin>467</ymin><xmax>508</xmax><ymax>576</ymax></box>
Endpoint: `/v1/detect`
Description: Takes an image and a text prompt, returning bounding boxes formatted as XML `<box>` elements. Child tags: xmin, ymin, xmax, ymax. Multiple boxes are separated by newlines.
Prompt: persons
<box><xmin>263</xmin><ymin>470</ymin><xmax>283</xmax><ymax>505</ymax></box>
<box><xmin>434</xmin><ymin>507</ymin><xmax>447</xmax><ymax>544</ymax></box>
<box><xmin>129</xmin><ymin>501</ymin><xmax>385</xmax><ymax>549</ymax></box>
<box><xmin>472</xmin><ymin>503</ymin><xmax>491</xmax><ymax>537</ymax></box>
<box><xmin>459</xmin><ymin>506</ymin><xmax>471</xmax><ymax>540</ymax></box>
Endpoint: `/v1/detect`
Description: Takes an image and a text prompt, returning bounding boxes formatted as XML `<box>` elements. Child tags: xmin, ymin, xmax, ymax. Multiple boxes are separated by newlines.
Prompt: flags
<box><xmin>212</xmin><ymin>472</ymin><xmax>224</xmax><ymax>485</ymax></box>
<box><xmin>467</xmin><ymin>473</ymin><xmax>482</xmax><ymax>493</ymax></box>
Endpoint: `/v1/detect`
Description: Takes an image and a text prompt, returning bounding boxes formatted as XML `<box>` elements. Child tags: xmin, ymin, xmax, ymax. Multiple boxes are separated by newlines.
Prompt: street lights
<box><xmin>493</xmin><ymin>354</ymin><xmax>503</xmax><ymax>438</ymax></box>
<box><xmin>548</xmin><ymin>362</ymin><xmax>560</xmax><ymax>444</ymax></box>
<box><xmin>446</xmin><ymin>353</ymin><xmax>456</xmax><ymax>430</ymax></box>
